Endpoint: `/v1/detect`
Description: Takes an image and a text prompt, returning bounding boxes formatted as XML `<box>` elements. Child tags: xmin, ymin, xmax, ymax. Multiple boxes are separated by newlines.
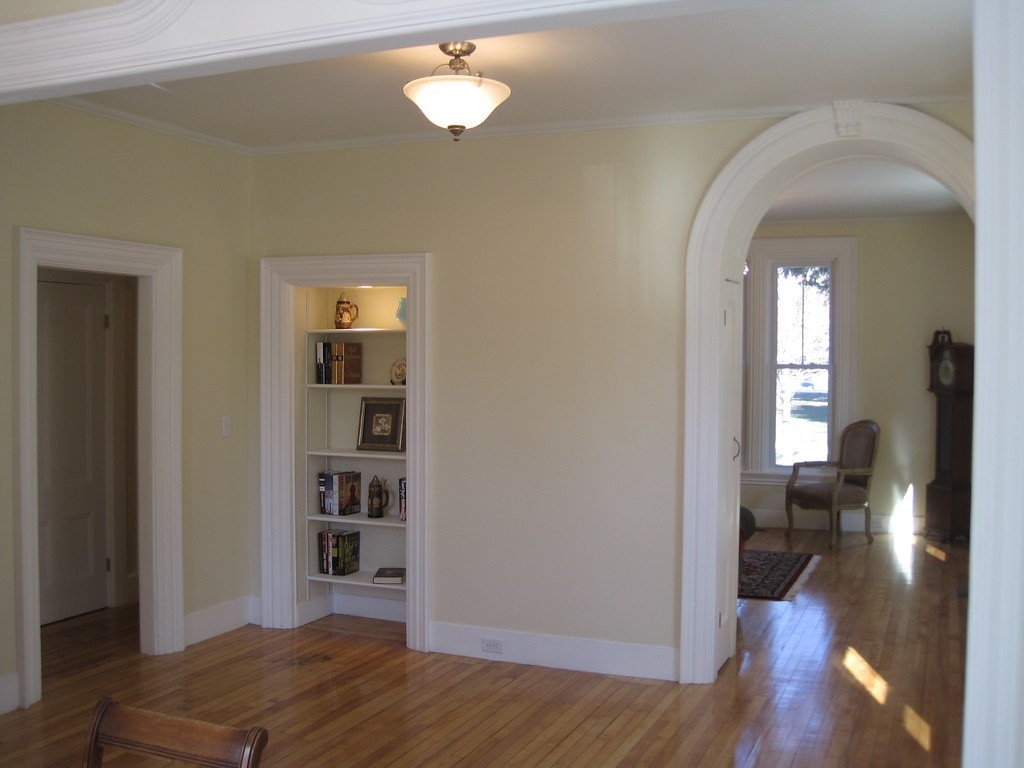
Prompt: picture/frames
<box><xmin>357</xmin><ymin>396</ymin><xmax>405</xmax><ymax>451</ymax></box>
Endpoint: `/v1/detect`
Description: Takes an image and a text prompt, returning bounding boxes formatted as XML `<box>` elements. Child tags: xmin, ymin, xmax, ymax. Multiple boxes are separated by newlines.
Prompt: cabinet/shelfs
<box><xmin>302</xmin><ymin>330</ymin><xmax>412</xmax><ymax>590</ymax></box>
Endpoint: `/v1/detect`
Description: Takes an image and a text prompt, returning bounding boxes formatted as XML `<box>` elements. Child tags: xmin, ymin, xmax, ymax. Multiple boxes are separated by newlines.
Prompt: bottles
<box><xmin>367</xmin><ymin>475</ymin><xmax>385</xmax><ymax>519</ymax></box>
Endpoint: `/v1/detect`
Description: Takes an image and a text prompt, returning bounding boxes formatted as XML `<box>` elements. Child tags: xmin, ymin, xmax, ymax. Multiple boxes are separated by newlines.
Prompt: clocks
<box><xmin>937</xmin><ymin>359</ymin><xmax>956</xmax><ymax>388</ymax></box>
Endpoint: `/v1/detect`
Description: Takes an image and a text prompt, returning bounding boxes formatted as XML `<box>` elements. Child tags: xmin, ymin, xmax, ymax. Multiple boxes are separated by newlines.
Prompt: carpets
<box><xmin>738</xmin><ymin>549</ymin><xmax>821</xmax><ymax>601</ymax></box>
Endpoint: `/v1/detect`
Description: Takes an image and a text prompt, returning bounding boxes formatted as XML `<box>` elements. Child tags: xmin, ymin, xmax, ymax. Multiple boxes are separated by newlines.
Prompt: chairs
<box><xmin>785</xmin><ymin>419</ymin><xmax>880</xmax><ymax>550</ymax></box>
<box><xmin>76</xmin><ymin>696</ymin><xmax>268</xmax><ymax>768</ymax></box>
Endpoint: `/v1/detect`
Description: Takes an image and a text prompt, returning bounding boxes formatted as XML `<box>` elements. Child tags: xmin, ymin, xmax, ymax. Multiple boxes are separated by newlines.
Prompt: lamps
<box><xmin>402</xmin><ymin>42</ymin><xmax>511</xmax><ymax>142</ymax></box>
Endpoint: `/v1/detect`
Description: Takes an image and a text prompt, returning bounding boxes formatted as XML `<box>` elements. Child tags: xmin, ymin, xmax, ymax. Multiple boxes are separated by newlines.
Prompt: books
<box><xmin>399</xmin><ymin>478</ymin><xmax>406</xmax><ymax>521</ymax></box>
<box><xmin>317</xmin><ymin>342</ymin><xmax>361</xmax><ymax>385</ymax></box>
<box><xmin>373</xmin><ymin>568</ymin><xmax>406</xmax><ymax>583</ymax></box>
<box><xmin>318</xmin><ymin>471</ymin><xmax>361</xmax><ymax>515</ymax></box>
<box><xmin>318</xmin><ymin>529</ymin><xmax>360</xmax><ymax>576</ymax></box>
